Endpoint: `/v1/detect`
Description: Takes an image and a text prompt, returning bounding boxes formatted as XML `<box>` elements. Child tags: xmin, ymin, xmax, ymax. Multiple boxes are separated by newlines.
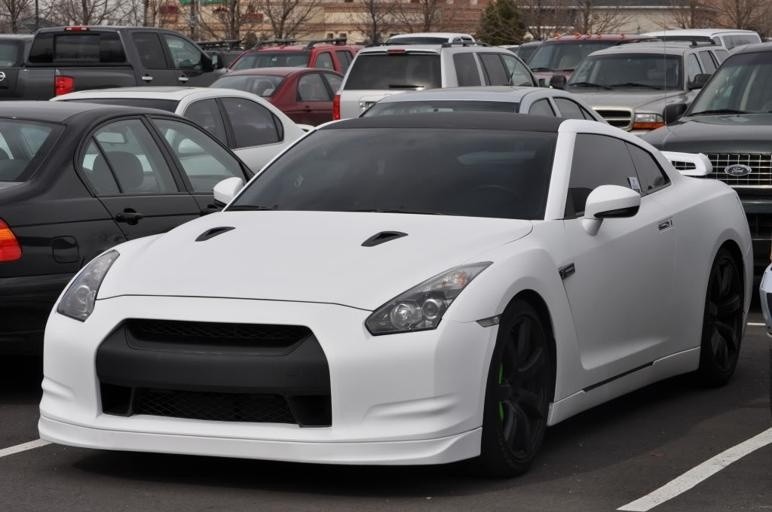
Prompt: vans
<box><xmin>331</xmin><ymin>46</ymin><xmax>555</xmax><ymax>124</ymax></box>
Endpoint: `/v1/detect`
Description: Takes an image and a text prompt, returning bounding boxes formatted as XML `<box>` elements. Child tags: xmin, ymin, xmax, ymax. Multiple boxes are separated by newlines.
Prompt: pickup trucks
<box><xmin>0</xmin><ymin>25</ymin><xmax>228</xmax><ymax>105</ymax></box>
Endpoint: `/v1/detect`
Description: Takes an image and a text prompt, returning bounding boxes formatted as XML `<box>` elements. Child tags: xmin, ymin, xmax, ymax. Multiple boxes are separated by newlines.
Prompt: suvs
<box><xmin>638</xmin><ymin>43</ymin><xmax>772</xmax><ymax>310</ymax></box>
<box><xmin>561</xmin><ymin>43</ymin><xmax>737</xmax><ymax>140</ymax></box>
<box><xmin>519</xmin><ymin>35</ymin><xmax>659</xmax><ymax>91</ymax></box>
<box><xmin>377</xmin><ymin>33</ymin><xmax>477</xmax><ymax>48</ymax></box>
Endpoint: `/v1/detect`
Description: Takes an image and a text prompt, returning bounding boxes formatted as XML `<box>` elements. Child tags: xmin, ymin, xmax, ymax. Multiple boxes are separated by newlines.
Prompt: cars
<box><xmin>37</xmin><ymin>112</ymin><xmax>757</xmax><ymax>481</ymax></box>
<box><xmin>46</xmin><ymin>83</ymin><xmax>317</xmax><ymax>179</ymax></box>
<box><xmin>0</xmin><ymin>28</ymin><xmax>35</xmax><ymax>100</ymax></box>
<box><xmin>221</xmin><ymin>46</ymin><xmax>364</xmax><ymax>80</ymax></box>
<box><xmin>206</xmin><ymin>65</ymin><xmax>346</xmax><ymax>129</ymax></box>
<box><xmin>0</xmin><ymin>99</ymin><xmax>256</xmax><ymax>398</ymax></box>
